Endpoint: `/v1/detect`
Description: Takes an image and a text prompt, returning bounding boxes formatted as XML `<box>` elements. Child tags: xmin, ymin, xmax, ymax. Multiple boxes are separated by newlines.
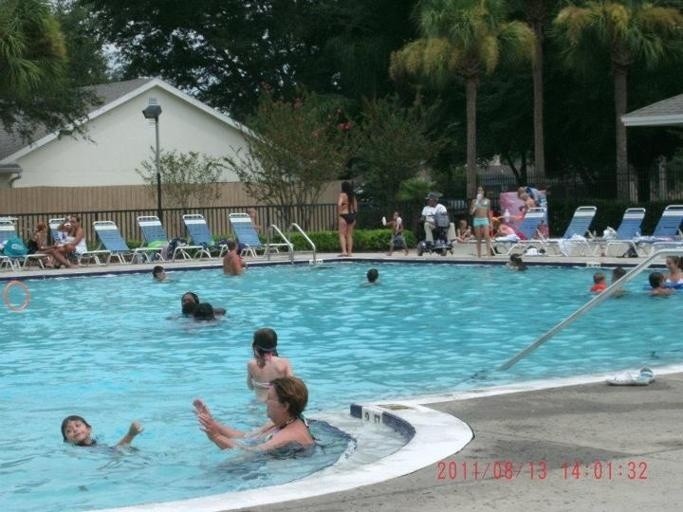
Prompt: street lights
<box><xmin>142</xmin><ymin>105</ymin><xmax>161</xmax><ymax>242</ymax></box>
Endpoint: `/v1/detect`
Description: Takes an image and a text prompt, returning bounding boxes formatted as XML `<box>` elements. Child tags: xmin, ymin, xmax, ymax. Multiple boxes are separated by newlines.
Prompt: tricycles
<box><xmin>415</xmin><ymin>211</ymin><xmax>452</xmax><ymax>255</ymax></box>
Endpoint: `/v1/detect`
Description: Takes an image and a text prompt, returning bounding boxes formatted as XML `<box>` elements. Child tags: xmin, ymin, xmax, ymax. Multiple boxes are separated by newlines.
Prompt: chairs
<box><xmin>542</xmin><ymin>206</ymin><xmax>598</xmax><ymax>259</ymax></box>
<box><xmin>590</xmin><ymin>207</ymin><xmax>647</xmax><ymax>258</ymax></box>
<box><xmin>91</xmin><ymin>220</ymin><xmax>165</xmax><ymax>264</ymax></box>
<box><xmin>484</xmin><ymin>206</ymin><xmax>549</xmax><ymax>259</ymax></box>
<box><xmin>46</xmin><ymin>217</ymin><xmax>111</xmax><ymax>267</ymax></box>
<box><xmin>635</xmin><ymin>204</ymin><xmax>682</xmax><ymax>256</ymax></box>
<box><xmin>181</xmin><ymin>213</ymin><xmax>255</xmax><ymax>259</ymax></box>
<box><xmin>227</xmin><ymin>212</ymin><xmax>293</xmax><ymax>260</ymax></box>
<box><xmin>135</xmin><ymin>215</ymin><xmax>211</xmax><ymax>263</ymax></box>
<box><xmin>0</xmin><ymin>219</ymin><xmax>48</xmax><ymax>272</ymax></box>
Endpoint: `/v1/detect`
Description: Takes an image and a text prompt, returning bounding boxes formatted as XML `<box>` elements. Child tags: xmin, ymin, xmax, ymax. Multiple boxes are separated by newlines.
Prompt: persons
<box><xmin>467</xmin><ymin>185</ymin><xmax>492</xmax><ymax>259</ymax></box>
<box><xmin>223</xmin><ymin>238</ymin><xmax>246</xmax><ymax>274</ymax></box>
<box><xmin>153</xmin><ymin>266</ymin><xmax>166</xmax><ymax>281</ymax></box>
<box><xmin>591</xmin><ymin>255</ymin><xmax>682</xmax><ymax>294</ymax></box>
<box><xmin>420</xmin><ymin>193</ymin><xmax>457</xmax><ymax>251</ymax></box>
<box><xmin>456</xmin><ymin>219</ymin><xmax>549</xmax><ymax>271</ymax></box>
<box><xmin>367</xmin><ymin>269</ymin><xmax>378</xmax><ymax>282</ymax></box>
<box><xmin>28</xmin><ymin>215</ymin><xmax>86</xmax><ymax>269</ymax></box>
<box><xmin>336</xmin><ymin>180</ymin><xmax>358</xmax><ymax>257</ymax></box>
<box><xmin>59</xmin><ymin>415</ymin><xmax>145</xmax><ymax>456</ymax></box>
<box><xmin>246</xmin><ymin>329</ymin><xmax>292</xmax><ymax>403</ymax></box>
<box><xmin>182</xmin><ymin>292</ymin><xmax>215</xmax><ymax>321</ymax></box>
<box><xmin>385</xmin><ymin>211</ymin><xmax>408</xmax><ymax>256</ymax></box>
<box><xmin>518</xmin><ymin>183</ymin><xmax>541</xmax><ymax>212</ymax></box>
<box><xmin>189</xmin><ymin>377</ymin><xmax>315</xmax><ymax>479</ymax></box>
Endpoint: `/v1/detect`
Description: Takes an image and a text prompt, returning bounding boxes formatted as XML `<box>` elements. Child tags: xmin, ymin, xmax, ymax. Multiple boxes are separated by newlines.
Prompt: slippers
<box><xmin>607</xmin><ymin>367</ymin><xmax>655</xmax><ymax>385</ymax></box>
